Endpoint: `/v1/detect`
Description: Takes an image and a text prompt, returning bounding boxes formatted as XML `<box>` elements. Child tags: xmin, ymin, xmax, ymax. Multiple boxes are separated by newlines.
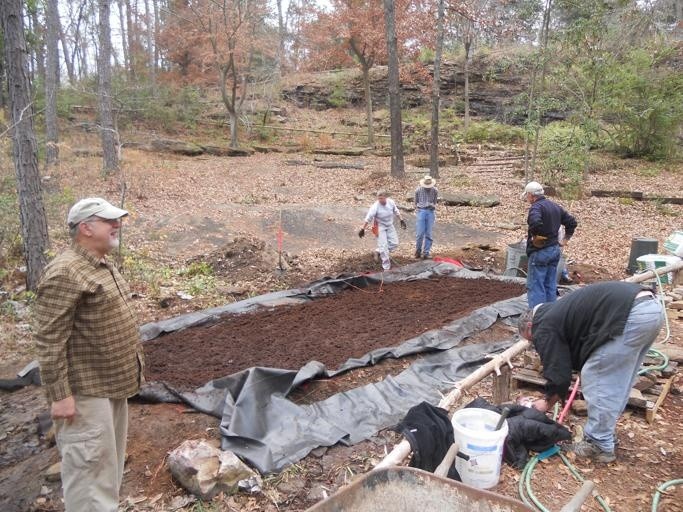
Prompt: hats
<box><xmin>522</xmin><ymin>182</ymin><xmax>543</xmax><ymax>196</ymax></box>
<box><xmin>420</xmin><ymin>176</ymin><xmax>436</xmax><ymax>189</ymax></box>
<box><xmin>67</xmin><ymin>197</ymin><xmax>130</xmax><ymax>228</ymax></box>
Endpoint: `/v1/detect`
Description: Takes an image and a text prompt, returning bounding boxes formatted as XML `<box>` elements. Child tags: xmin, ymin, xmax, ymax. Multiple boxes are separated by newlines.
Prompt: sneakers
<box><xmin>562</xmin><ymin>439</ymin><xmax>616</xmax><ymax>462</ymax></box>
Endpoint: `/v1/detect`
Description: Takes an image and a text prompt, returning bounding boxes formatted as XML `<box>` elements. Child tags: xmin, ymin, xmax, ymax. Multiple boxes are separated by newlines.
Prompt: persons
<box><xmin>358</xmin><ymin>189</ymin><xmax>407</xmax><ymax>270</ymax></box>
<box><xmin>521</xmin><ymin>181</ymin><xmax>576</xmax><ymax>308</ymax></box>
<box><xmin>32</xmin><ymin>196</ymin><xmax>144</xmax><ymax>512</ymax></box>
<box><xmin>558</xmin><ymin>224</ymin><xmax>572</xmax><ymax>283</ymax></box>
<box><xmin>413</xmin><ymin>176</ymin><xmax>438</xmax><ymax>259</ymax></box>
<box><xmin>518</xmin><ymin>279</ymin><xmax>667</xmax><ymax>464</ymax></box>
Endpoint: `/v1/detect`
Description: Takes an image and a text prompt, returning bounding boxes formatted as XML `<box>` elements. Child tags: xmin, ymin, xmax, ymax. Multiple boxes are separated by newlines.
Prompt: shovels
<box><xmin>277</xmin><ymin>208</ymin><xmax>286</xmax><ymax>273</ymax></box>
<box><xmin>118</xmin><ymin>181</ymin><xmax>126</xmax><ymax>276</ymax></box>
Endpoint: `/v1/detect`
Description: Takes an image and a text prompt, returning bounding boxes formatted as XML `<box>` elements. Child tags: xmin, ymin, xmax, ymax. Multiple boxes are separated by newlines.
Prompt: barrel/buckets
<box><xmin>625</xmin><ymin>236</ymin><xmax>658</xmax><ymax>277</ymax></box>
<box><xmin>507</xmin><ymin>241</ymin><xmax>526</xmax><ymax>276</ymax></box>
<box><xmin>452</xmin><ymin>407</ymin><xmax>508</xmax><ymax>489</ymax></box>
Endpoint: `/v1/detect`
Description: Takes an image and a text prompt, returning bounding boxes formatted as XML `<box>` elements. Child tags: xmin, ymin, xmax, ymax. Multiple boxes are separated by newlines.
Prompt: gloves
<box><xmin>359</xmin><ymin>229</ymin><xmax>364</xmax><ymax>239</ymax></box>
<box><xmin>401</xmin><ymin>220</ymin><xmax>407</xmax><ymax>229</ymax></box>
